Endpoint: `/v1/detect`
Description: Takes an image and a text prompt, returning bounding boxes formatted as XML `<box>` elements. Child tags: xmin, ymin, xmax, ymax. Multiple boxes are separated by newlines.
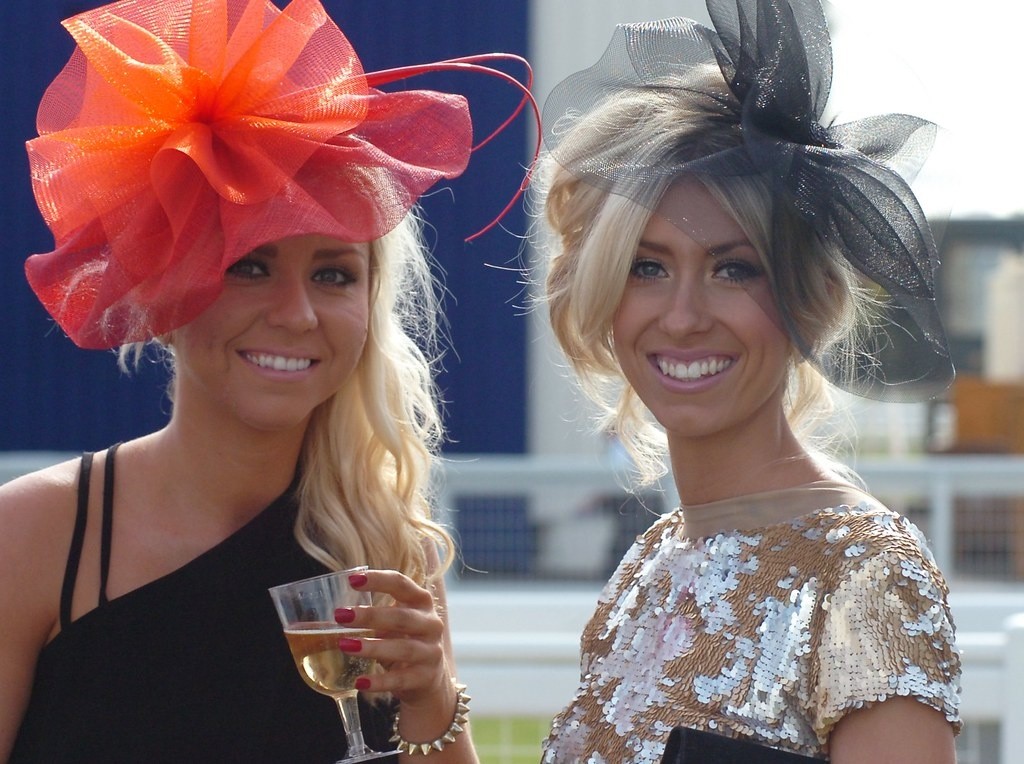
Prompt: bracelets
<box><xmin>391</xmin><ymin>677</ymin><xmax>471</xmax><ymax>755</ymax></box>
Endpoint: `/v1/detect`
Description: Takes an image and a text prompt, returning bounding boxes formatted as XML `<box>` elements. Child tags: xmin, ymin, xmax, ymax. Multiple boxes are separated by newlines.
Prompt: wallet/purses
<box><xmin>660</xmin><ymin>727</ymin><xmax>831</xmax><ymax>764</ymax></box>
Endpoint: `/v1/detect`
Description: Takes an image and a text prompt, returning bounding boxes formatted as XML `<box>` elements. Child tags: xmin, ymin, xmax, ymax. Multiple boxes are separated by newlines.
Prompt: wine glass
<box><xmin>268</xmin><ymin>566</ymin><xmax>402</xmax><ymax>764</ymax></box>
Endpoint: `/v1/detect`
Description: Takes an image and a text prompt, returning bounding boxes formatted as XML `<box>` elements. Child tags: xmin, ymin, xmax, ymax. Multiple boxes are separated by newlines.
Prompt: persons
<box><xmin>0</xmin><ymin>1</ymin><xmax>542</xmax><ymax>764</ymax></box>
<box><xmin>541</xmin><ymin>0</ymin><xmax>964</xmax><ymax>764</ymax></box>
